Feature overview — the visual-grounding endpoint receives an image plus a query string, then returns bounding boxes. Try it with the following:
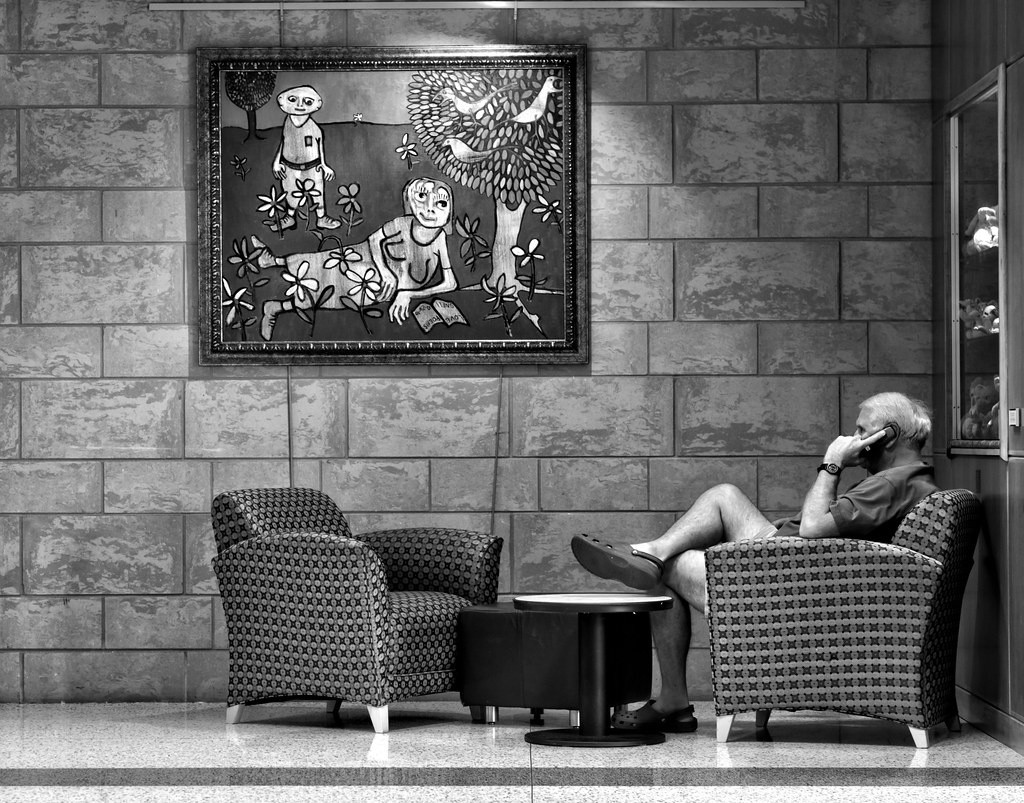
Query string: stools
[456,602,653,728]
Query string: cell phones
[858,427,895,459]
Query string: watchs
[816,462,841,475]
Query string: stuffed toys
[959,204,1012,441]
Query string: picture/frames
[195,43,591,367]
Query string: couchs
[211,487,504,735]
[705,489,982,749]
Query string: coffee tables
[512,593,674,748]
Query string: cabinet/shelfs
[936,62,1010,462]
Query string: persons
[572,392,937,733]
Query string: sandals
[571,532,664,590]
[611,699,698,732]
